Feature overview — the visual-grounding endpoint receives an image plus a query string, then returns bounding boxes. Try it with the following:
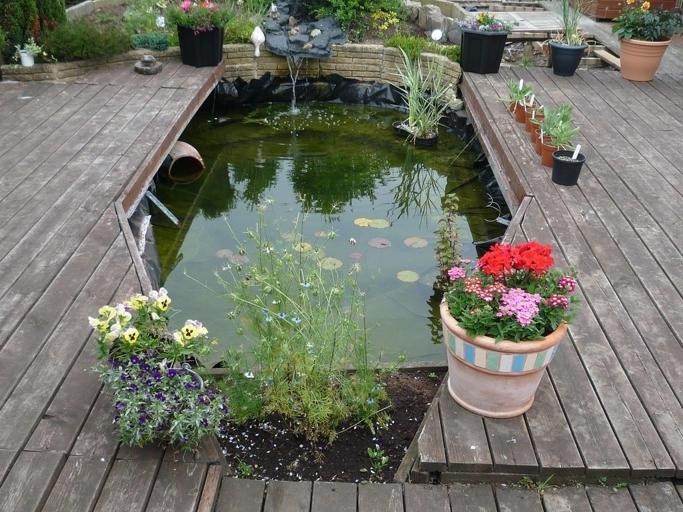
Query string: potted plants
[390,47,456,146]
[550,0,588,76]
[508,80,585,185]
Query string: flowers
[612,0,683,39]
[448,242,578,343]
[86,287,226,450]
[167,0,236,29]
[14,37,43,56]
[462,12,513,32]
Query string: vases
[439,296,566,419]
[177,24,224,67]
[461,30,508,74]
[620,37,672,81]
[19,50,34,67]
